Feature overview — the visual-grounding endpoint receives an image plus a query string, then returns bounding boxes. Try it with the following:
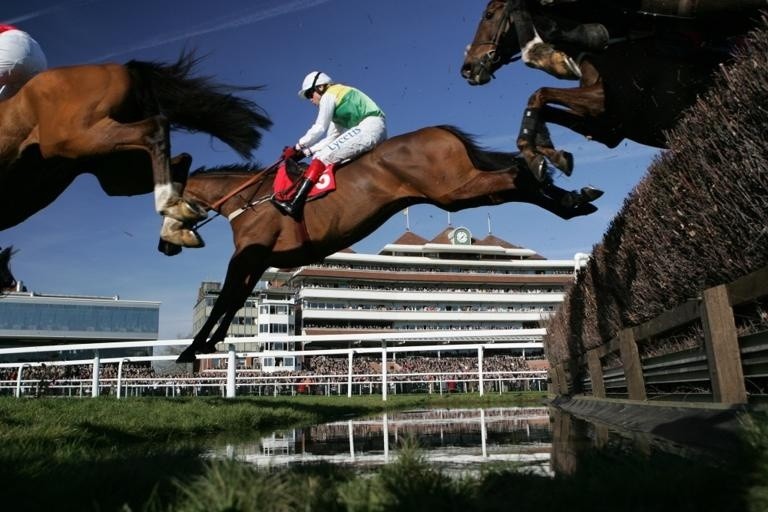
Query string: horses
[0,40,273,301]
[459,0,768,184]
[157,123,606,364]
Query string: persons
[0,20,49,97]
[269,70,387,224]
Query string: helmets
[297,71,333,98]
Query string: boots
[271,178,314,222]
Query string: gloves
[281,145,306,162]
